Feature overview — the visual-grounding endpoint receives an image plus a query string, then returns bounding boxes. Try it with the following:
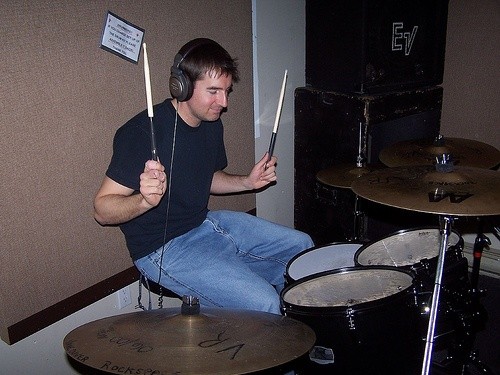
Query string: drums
[355,228,474,340]
[280,266,423,375]
[283,241,366,288]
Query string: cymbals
[318,163,386,189]
[64,296,317,375]
[352,167,500,216]
[378,135,500,168]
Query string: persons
[94,38,314,314]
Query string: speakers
[293,83,444,248]
[304,0,446,96]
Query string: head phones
[168,40,209,102]
